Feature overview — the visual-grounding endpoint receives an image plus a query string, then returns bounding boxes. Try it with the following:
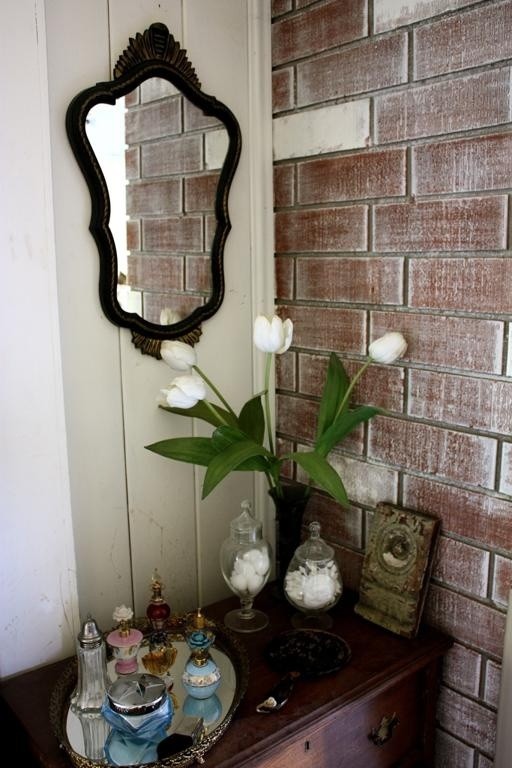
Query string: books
[354,500,441,640]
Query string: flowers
[149,318,422,498]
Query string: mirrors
[64,24,242,361]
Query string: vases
[265,484,310,594]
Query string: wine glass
[217,500,273,633]
[284,520,344,631]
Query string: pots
[255,629,351,716]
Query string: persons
[382,536,407,568]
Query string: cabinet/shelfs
[0,575,452,768]
